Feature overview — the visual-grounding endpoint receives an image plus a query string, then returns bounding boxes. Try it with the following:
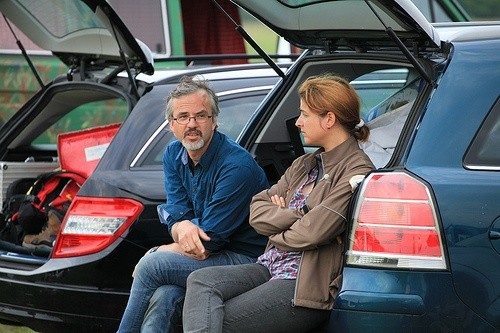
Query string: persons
[114,76,273,333]
[182,72,379,333]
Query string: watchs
[149,245,160,253]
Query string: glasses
[173,113,213,125]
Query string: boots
[22,209,63,249]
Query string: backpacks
[17,168,89,233]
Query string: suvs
[1,0,291,332]
[211,0,500,332]
